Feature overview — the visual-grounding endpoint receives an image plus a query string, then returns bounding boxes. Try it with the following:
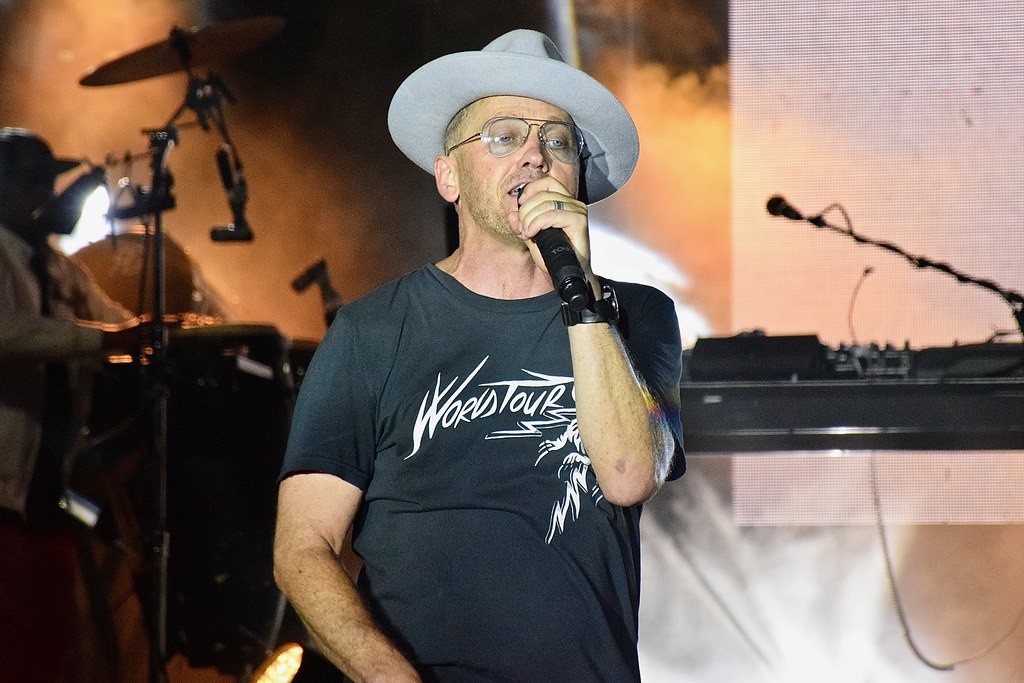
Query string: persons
[273,29,686,683]
[0,127,265,669]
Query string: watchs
[560,285,620,327]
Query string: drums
[65,230,227,328]
[159,322,295,454]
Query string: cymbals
[79,14,291,89]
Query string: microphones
[518,182,589,311]
[291,257,327,293]
[767,196,803,220]
[210,224,254,244]
[108,192,177,219]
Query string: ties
[30,256,51,318]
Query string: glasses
[447,115,585,164]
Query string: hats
[0,127,80,177]
[387,29,640,205]
